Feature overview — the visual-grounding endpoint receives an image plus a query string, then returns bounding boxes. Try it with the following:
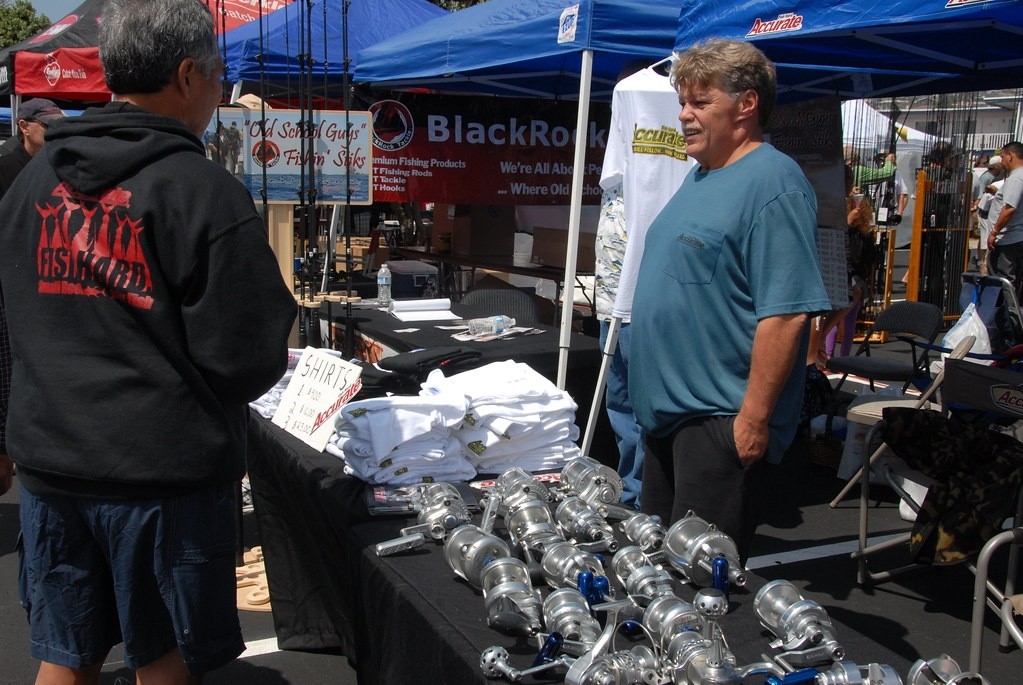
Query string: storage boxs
[531,227,597,272]
[337,242,389,272]
[452,203,516,256]
[384,260,438,298]
[837,399,953,489]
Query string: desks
[318,299,620,471]
[398,245,597,333]
[246,366,918,685]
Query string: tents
[579,0,1023,458]
[318,0,677,388]
[0,0,291,137]
[841,98,952,250]
[215,0,446,111]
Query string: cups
[854,194,864,208]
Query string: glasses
[25,119,49,129]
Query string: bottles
[377,264,391,305]
[470,314,516,335]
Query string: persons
[844,149,908,296]
[596,181,647,508]
[902,142,1023,305]
[0,0,297,685]
[0,99,66,494]
[628,42,833,569]
[203,122,244,177]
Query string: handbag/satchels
[929,284,993,374]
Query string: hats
[989,156,1002,165]
[15,99,65,126]
[843,146,858,165]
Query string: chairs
[463,288,536,321]
[824,273,1023,671]
